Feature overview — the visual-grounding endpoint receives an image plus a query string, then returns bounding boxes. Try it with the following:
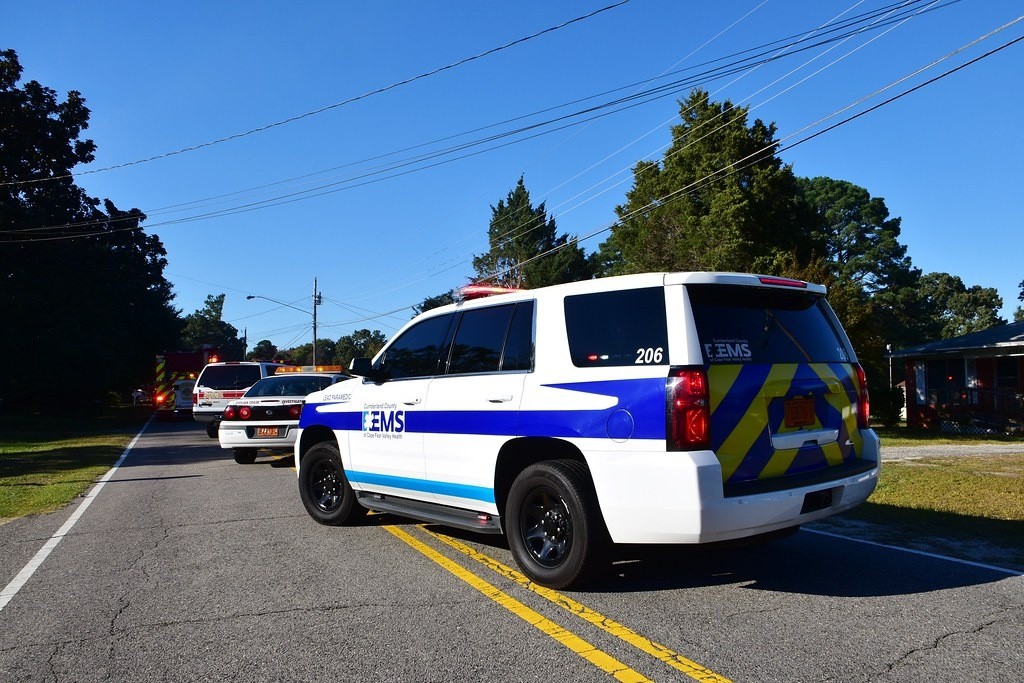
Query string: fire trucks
[146,343,224,423]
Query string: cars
[218,365,352,464]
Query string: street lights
[247,295,317,367]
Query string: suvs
[191,359,288,439]
[293,273,882,593]
